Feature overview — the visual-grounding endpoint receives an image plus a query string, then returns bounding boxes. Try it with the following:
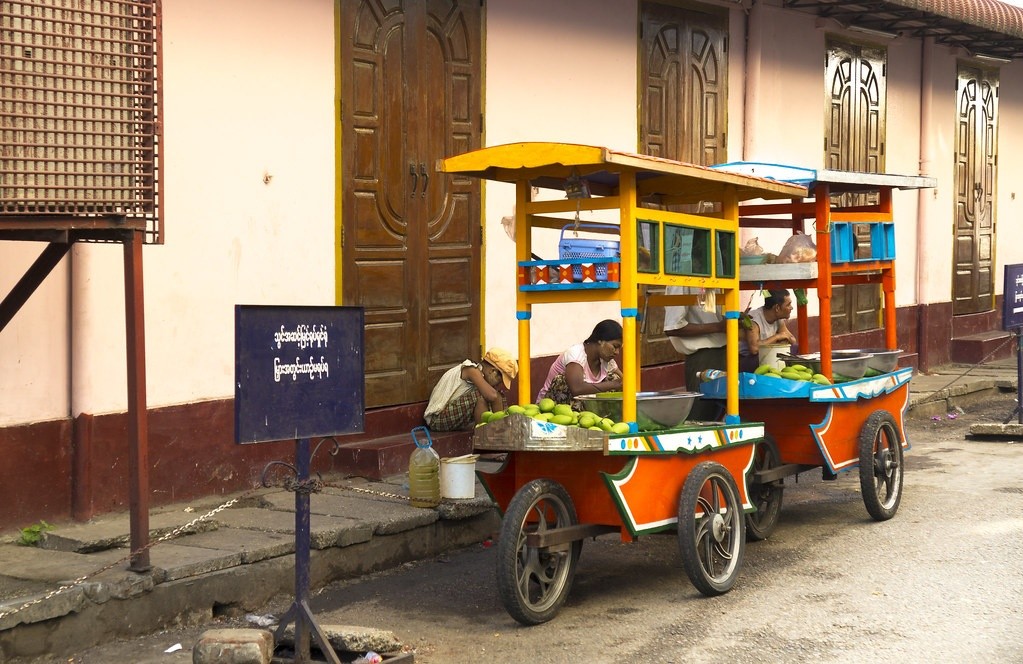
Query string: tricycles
[691,148,938,522]
[435,141,768,628]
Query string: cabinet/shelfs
[493,166,742,428]
[735,183,897,377]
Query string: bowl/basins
[829,348,905,377]
[738,254,768,266]
[573,391,704,432]
[784,351,874,384]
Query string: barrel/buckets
[440,457,476,499]
[757,344,791,373]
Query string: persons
[535,318,622,413]
[745,289,798,372]
[424,346,518,461]
[665,286,748,423]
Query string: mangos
[475,398,630,434]
[753,364,831,384]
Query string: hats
[483,346,518,390]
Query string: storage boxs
[558,224,622,281]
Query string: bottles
[409,426,441,508]
[696,369,727,382]
